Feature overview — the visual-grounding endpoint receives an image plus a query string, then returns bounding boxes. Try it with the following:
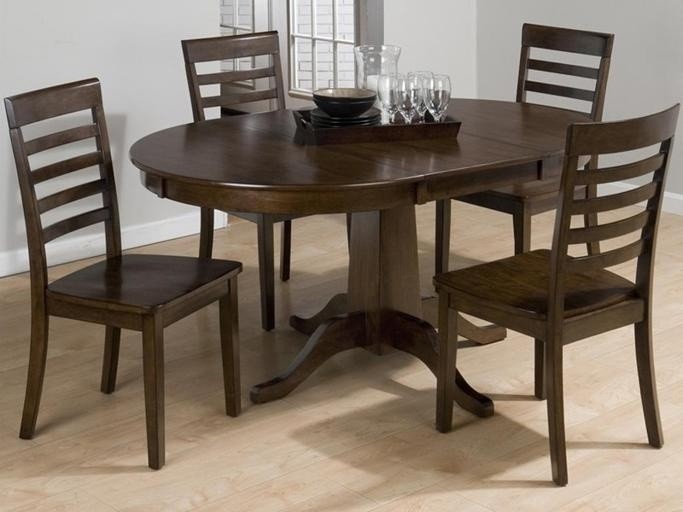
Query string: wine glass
[376,70,453,124]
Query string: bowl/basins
[311,87,378,118]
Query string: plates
[309,106,383,130]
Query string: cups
[353,45,402,127]
